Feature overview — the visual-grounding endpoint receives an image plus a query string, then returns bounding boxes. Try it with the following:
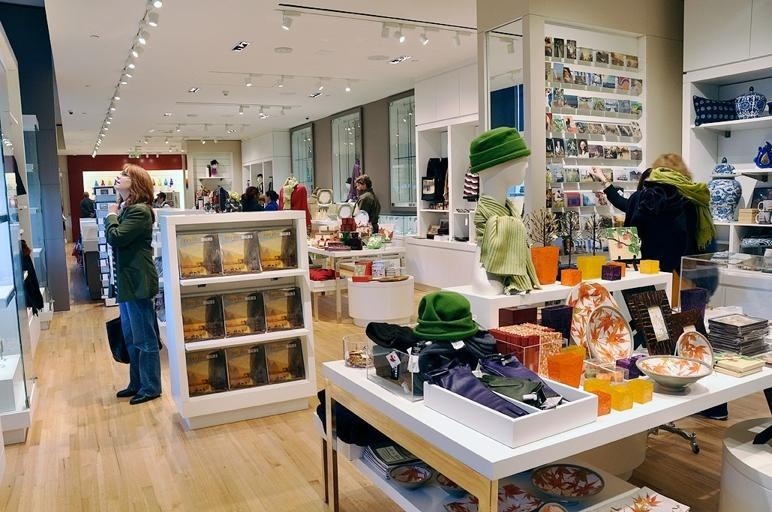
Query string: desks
[308,246,407,323]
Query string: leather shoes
[130,392,160,405]
[117,388,140,397]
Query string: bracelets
[601,178,609,185]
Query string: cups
[755,199,772,223]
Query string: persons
[263,190,281,211]
[467,125,541,298]
[554,139,565,158]
[80,191,97,218]
[622,152,728,421]
[587,165,652,227]
[276,176,312,227]
[257,173,263,193]
[561,68,572,83]
[353,172,380,237]
[240,186,264,212]
[345,178,354,204]
[576,139,588,159]
[102,161,163,406]
[154,190,170,210]
[553,189,563,204]
[210,159,220,178]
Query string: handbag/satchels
[106,312,162,364]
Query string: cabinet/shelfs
[682,0,772,322]
[402,63,479,293]
[0,23,54,512]
[159,209,318,430]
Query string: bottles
[95,179,117,186]
[206,165,211,178]
[152,178,174,188]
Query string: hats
[413,291,478,340]
[470,127,531,174]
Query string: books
[702,313,771,379]
[360,441,429,480]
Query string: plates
[566,279,620,348]
[637,354,714,393]
[531,461,607,502]
[586,305,634,366]
[435,473,467,500]
[588,485,692,512]
[438,482,539,512]
[388,464,432,491]
[539,501,569,512]
[676,331,715,371]
